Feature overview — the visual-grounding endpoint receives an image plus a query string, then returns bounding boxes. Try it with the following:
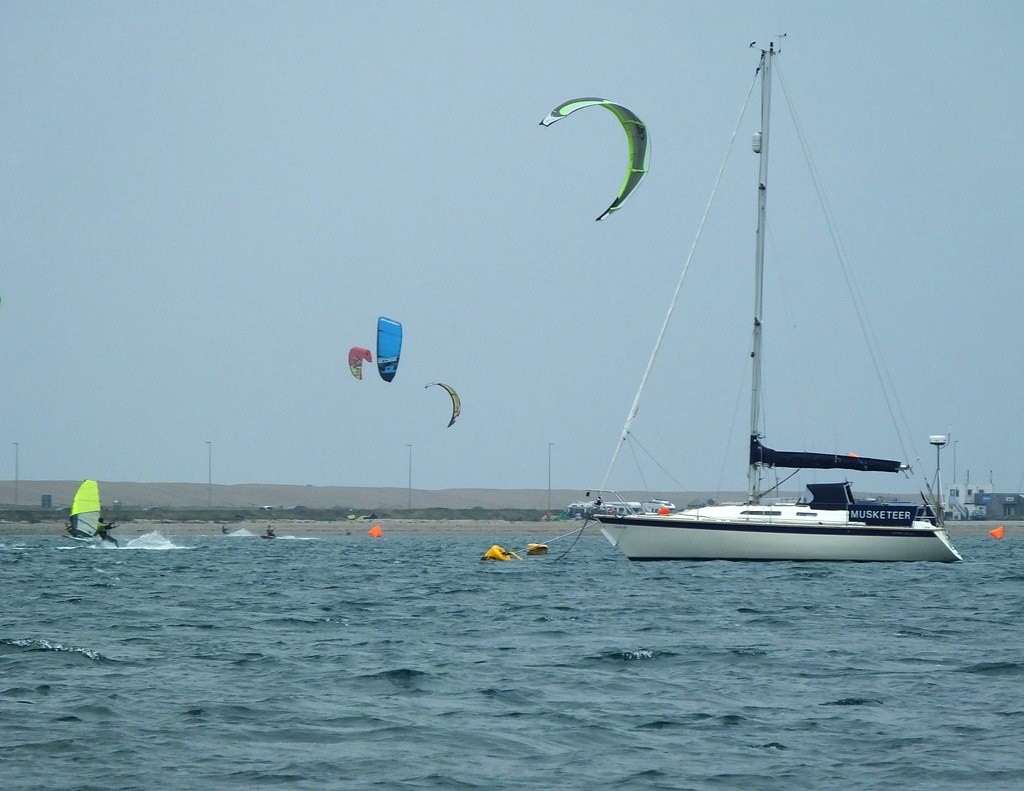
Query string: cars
[970,509,987,521]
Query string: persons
[97,517,120,548]
[267,525,276,536]
[222,525,229,534]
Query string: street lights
[407,444,411,516]
[11,442,18,505]
[547,442,555,518]
[205,441,211,510]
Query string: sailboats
[60,479,122,548]
[589,28,965,564]
